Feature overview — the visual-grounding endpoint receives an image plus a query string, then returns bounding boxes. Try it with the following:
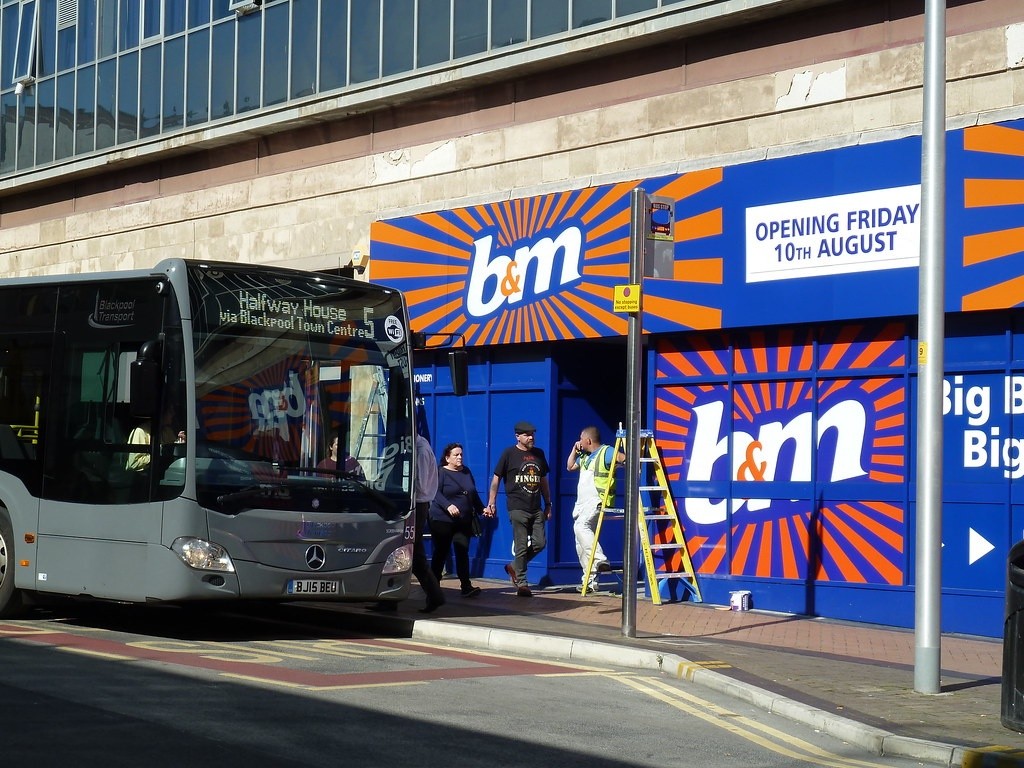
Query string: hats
[514,422,536,432]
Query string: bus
[0,256,469,620]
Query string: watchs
[546,502,552,507]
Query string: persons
[365,432,447,613]
[426,442,496,597]
[125,393,186,500]
[567,427,626,592]
[483,422,552,598]
[316,433,367,480]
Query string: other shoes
[365,600,397,612]
[461,586,481,597]
[518,586,532,597]
[418,601,444,613]
[577,585,593,593]
[504,564,519,587]
[599,564,613,575]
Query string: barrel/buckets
[729,590,751,611]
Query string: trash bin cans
[1001,539,1024,733]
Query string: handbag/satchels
[471,512,482,537]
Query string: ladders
[581,428,703,606]
